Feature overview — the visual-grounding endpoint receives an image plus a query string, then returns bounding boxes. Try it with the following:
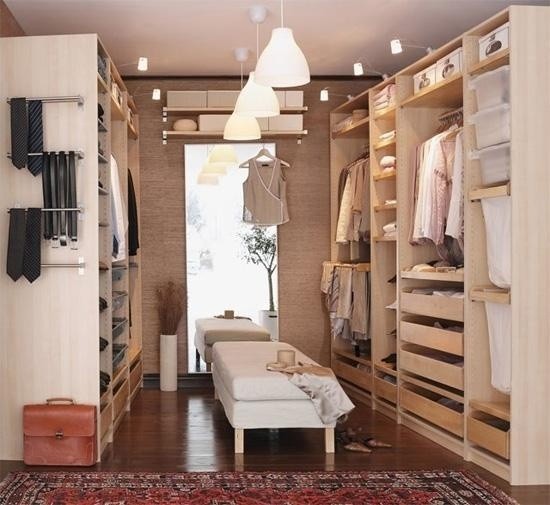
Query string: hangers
[238,144,292,171]
[346,145,368,166]
[413,107,463,148]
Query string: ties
[27,100,43,177]
[7,208,26,281]
[22,207,41,283]
[11,97,27,169]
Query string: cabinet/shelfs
[0,34,145,463]
[162,104,307,138]
[328,5,549,485]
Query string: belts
[42,151,51,246]
[50,151,59,248]
[59,151,67,246]
[68,151,78,250]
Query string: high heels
[345,426,392,447]
[335,426,372,452]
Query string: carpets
[0,471,523,504]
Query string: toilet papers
[278,350,295,366]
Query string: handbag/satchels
[23,397,98,467]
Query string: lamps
[116,57,148,74]
[233,6,281,118]
[353,63,386,79]
[132,88,161,101]
[320,88,352,101]
[254,0,311,88]
[390,39,434,54]
[223,48,262,139]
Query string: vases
[158,333,178,393]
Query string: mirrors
[183,144,279,374]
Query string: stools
[213,342,355,455]
[194,315,272,371]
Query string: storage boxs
[478,21,509,61]
[436,47,463,81]
[413,62,436,93]
[467,66,510,184]
